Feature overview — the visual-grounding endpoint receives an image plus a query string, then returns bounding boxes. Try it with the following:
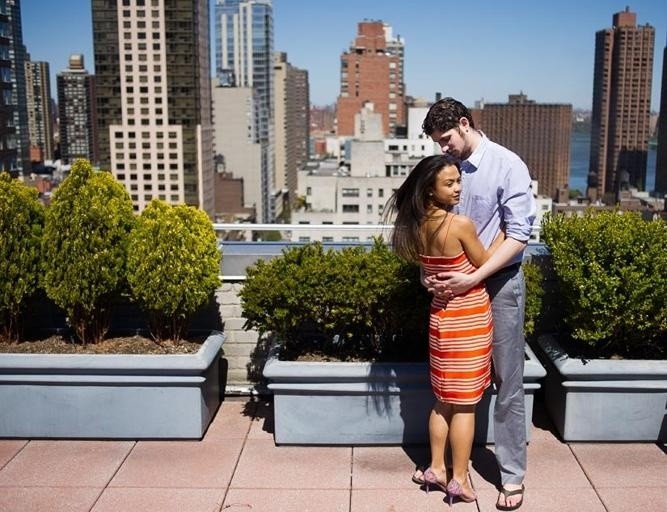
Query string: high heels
[446,478,477,507]
[423,467,452,494]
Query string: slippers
[412,464,430,484]
[496,484,525,511]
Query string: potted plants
[0,159,227,442]
[237,235,548,448]
[536,188,666,445]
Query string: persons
[377,153,504,505]
[421,95,537,511]
